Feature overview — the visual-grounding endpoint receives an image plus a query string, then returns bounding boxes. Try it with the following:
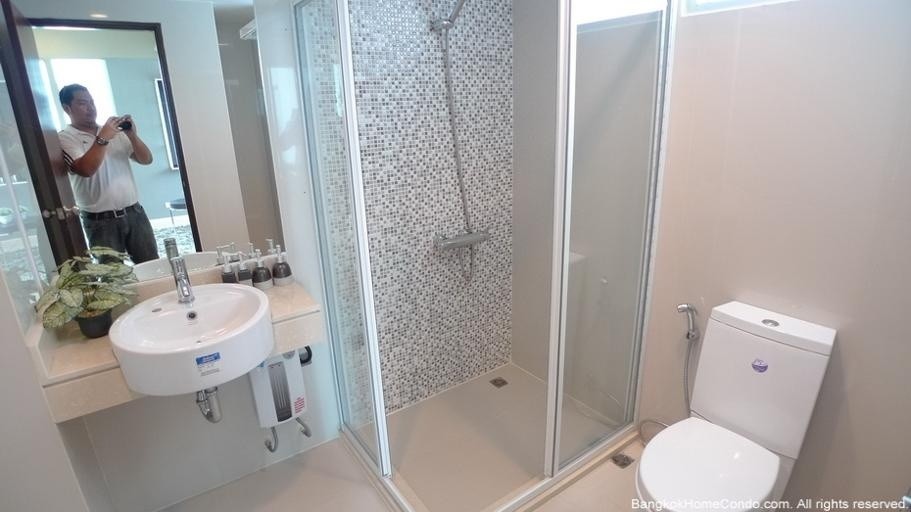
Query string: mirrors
[0,1,288,290]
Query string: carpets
[149,225,197,258]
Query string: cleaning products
[222,245,293,289]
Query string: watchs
[96,135,110,146]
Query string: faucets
[170,257,193,304]
[164,238,178,263]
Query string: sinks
[123,251,234,281]
[108,284,273,398]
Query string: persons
[56,84,161,266]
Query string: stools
[166,196,188,225]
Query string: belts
[80,204,142,221]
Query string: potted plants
[34,245,139,340]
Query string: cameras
[116,120,134,132]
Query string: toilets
[635,302,839,512]
